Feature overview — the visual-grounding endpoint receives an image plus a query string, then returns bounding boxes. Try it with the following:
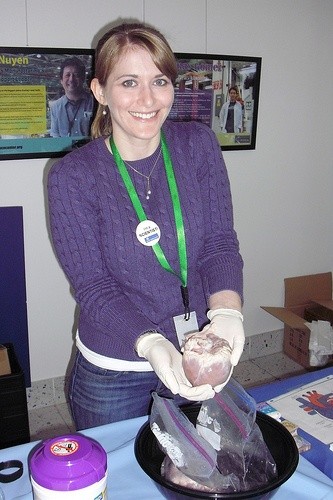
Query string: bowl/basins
[133,404,300,500]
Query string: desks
[0,368,332,500]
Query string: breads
[183,334,232,385]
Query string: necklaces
[123,149,162,200]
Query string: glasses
[63,72,82,78]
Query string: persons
[48,55,95,136]
[46,22,246,431]
[220,86,242,133]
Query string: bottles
[26,434,109,500]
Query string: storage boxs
[257,273,333,372]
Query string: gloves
[181,307,244,393]
[135,333,217,400]
